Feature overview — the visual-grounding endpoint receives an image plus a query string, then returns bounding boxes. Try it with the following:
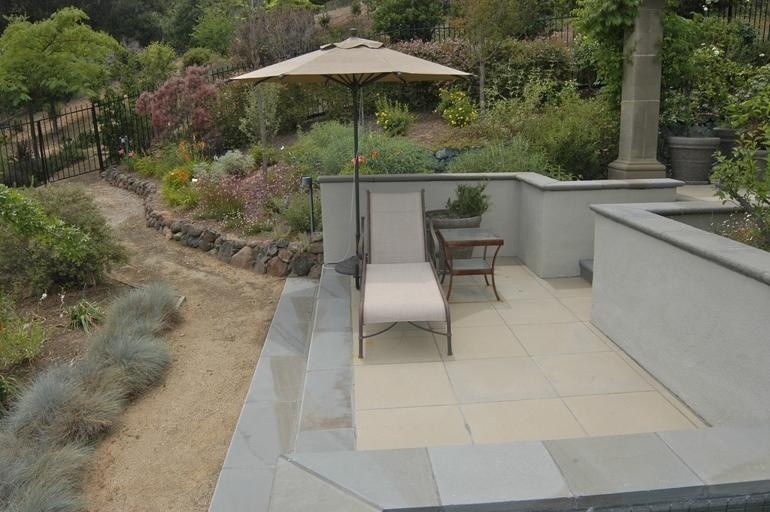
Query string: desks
[437,227,503,301]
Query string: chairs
[358,188,452,358]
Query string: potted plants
[426,180,493,274]
[666,92,770,184]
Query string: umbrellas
[223,27,479,289]
